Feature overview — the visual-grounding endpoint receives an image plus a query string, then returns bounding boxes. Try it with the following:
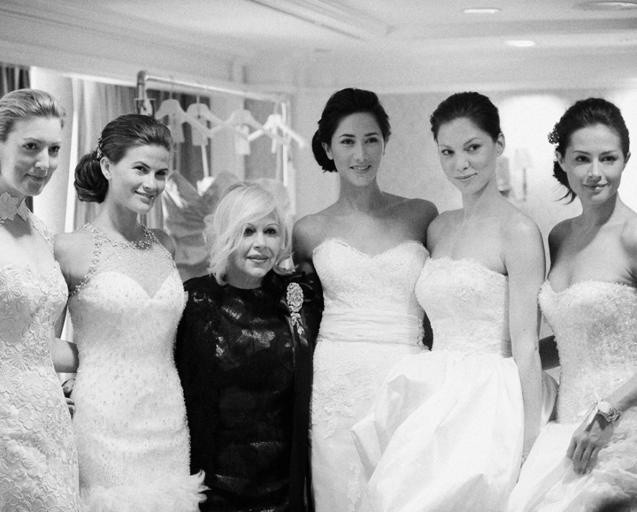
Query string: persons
[172,180,323,511]
[50,112,211,512]
[292,86,440,511]
[499,96,636,512]
[0,86,81,512]
[355,90,561,512]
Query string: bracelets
[592,396,622,424]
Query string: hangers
[152,102,307,160]
